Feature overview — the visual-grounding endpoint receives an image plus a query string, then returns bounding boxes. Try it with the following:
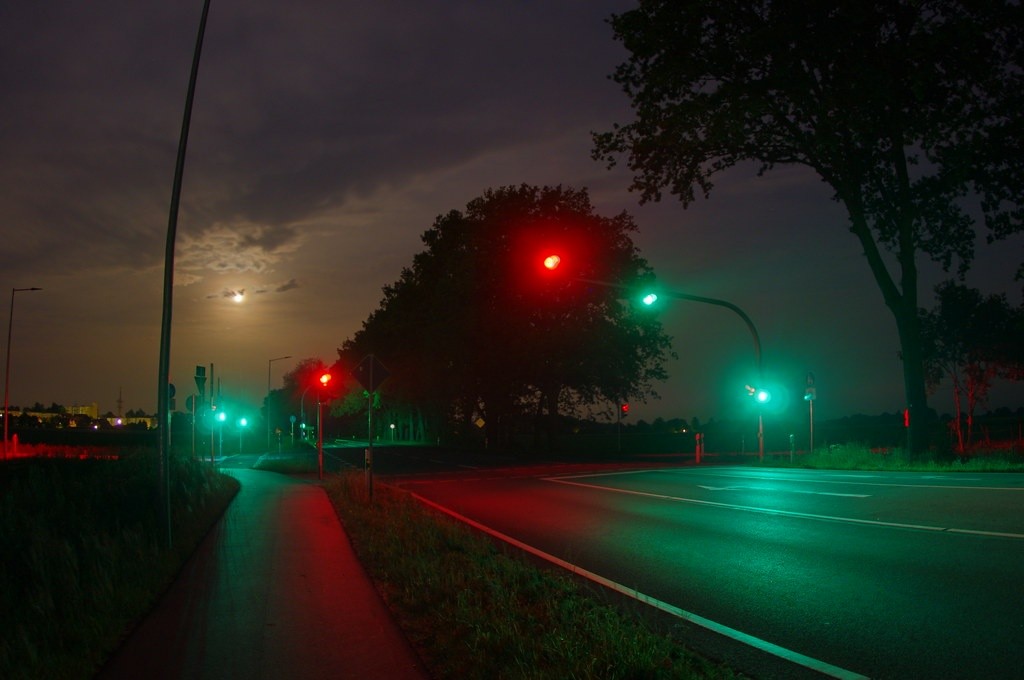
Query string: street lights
[3,286,45,461]
[267,355,293,453]
